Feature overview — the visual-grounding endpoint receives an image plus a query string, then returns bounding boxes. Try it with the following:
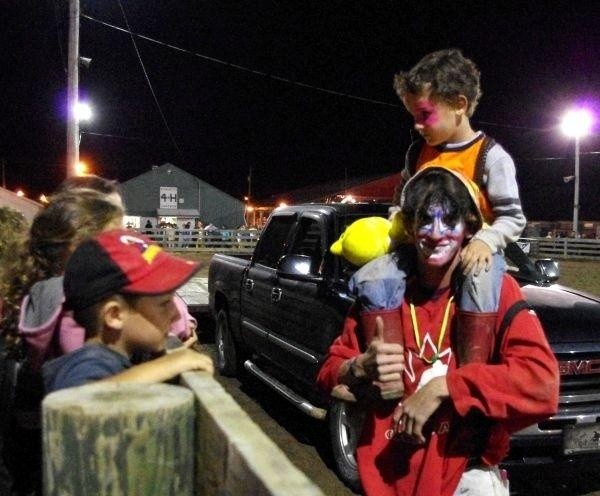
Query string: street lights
[561,105,592,238]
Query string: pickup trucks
[207,200,599,494]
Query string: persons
[6,231,207,493]
[62,175,123,208]
[18,193,198,356]
[348,50,527,401]
[317,166,559,496]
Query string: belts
[463,456,490,472]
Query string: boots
[356,308,406,402]
[453,308,500,370]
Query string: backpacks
[0,302,70,493]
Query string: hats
[60,227,202,312]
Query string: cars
[182,228,260,252]
